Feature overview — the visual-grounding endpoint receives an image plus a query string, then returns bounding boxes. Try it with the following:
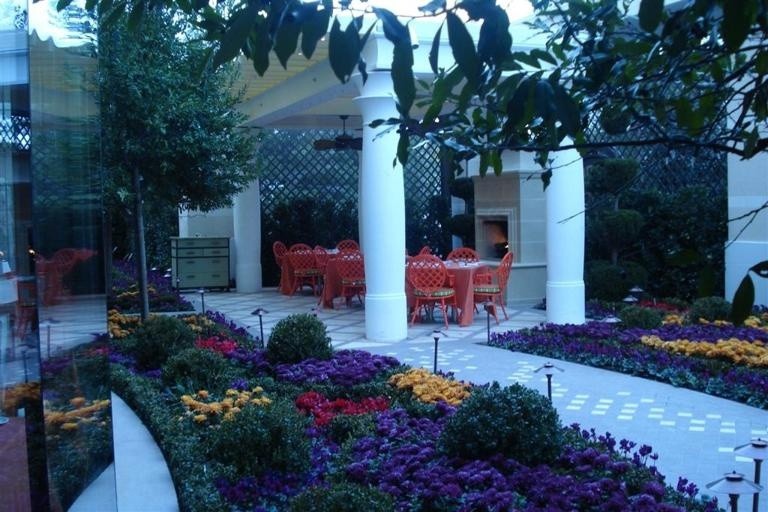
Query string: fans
[314,115,362,150]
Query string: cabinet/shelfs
[169,237,230,294]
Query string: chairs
[273,239,514,330]
[6,250,95,342]
